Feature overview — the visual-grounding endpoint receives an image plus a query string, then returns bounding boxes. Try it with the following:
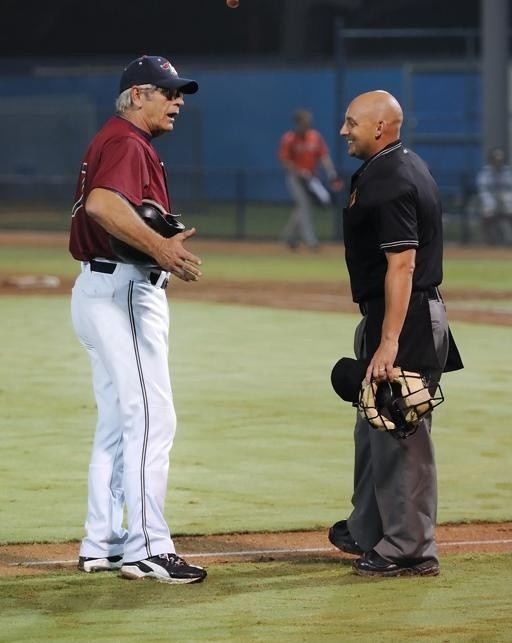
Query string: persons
[326,87,453,579]
[275,107,345,254]
[463,147,512,247]
[66,54,208,588]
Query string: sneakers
[328,519,369,555]
[78,550,125,573]
[122,555,209,586]
[353,542,440,580]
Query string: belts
[90,258,169,290]
[358,285,442,316]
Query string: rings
[379,368,386,372]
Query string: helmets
[358,365,445,440]
[108,199,185,266]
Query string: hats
[119,55,198,95]
